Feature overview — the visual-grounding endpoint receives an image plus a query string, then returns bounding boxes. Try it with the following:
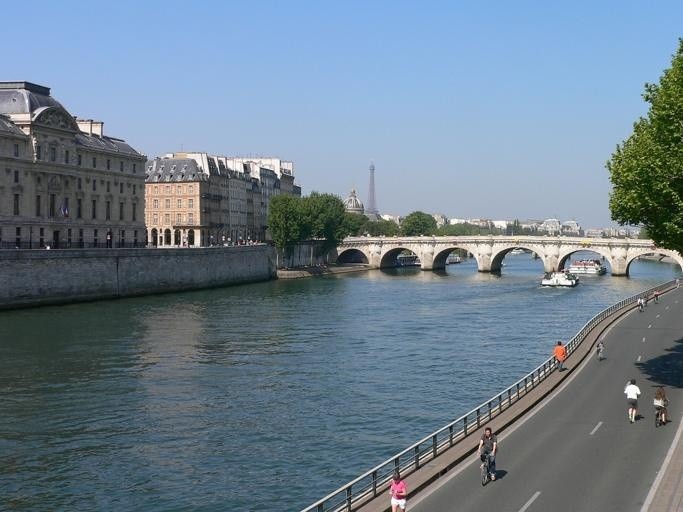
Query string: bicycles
[478,453,499,485]
[638,296,659,312]
[654,396,672,427]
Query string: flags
[60,205,71,218]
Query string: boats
[532,252,539,259]
[397,254,463,267]
[539,268,579,287]
[567,260,607,275]
[510,249,525,254]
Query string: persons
[389,471,407,512]
[597,340,605,362]
[637,288,662,312]
[208,233,260,247]
[676,278,680,289]
[553,341,568,372]
[653,386,669,425]
[624,379,642,424]
[476,427,497,481]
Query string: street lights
[105,232,112,249]
[209,239,262,247]
